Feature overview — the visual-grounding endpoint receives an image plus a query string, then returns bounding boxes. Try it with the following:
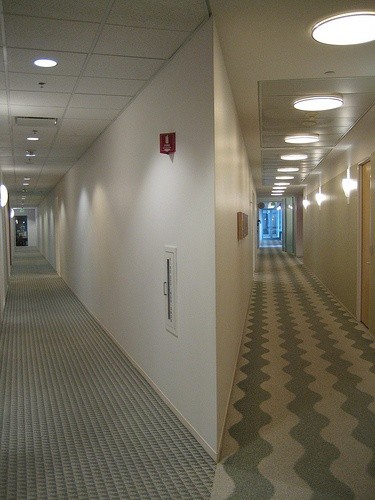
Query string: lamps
[10,208,14,218]
[285,133,319,144]
[303,196,310,208]
[271,168,299,195]
[342,169,358,204]
[280,154,308,160]
[315,187,326,206]
[312,10,375,45]
[0,185,8,207]
[293,95,343,111]
